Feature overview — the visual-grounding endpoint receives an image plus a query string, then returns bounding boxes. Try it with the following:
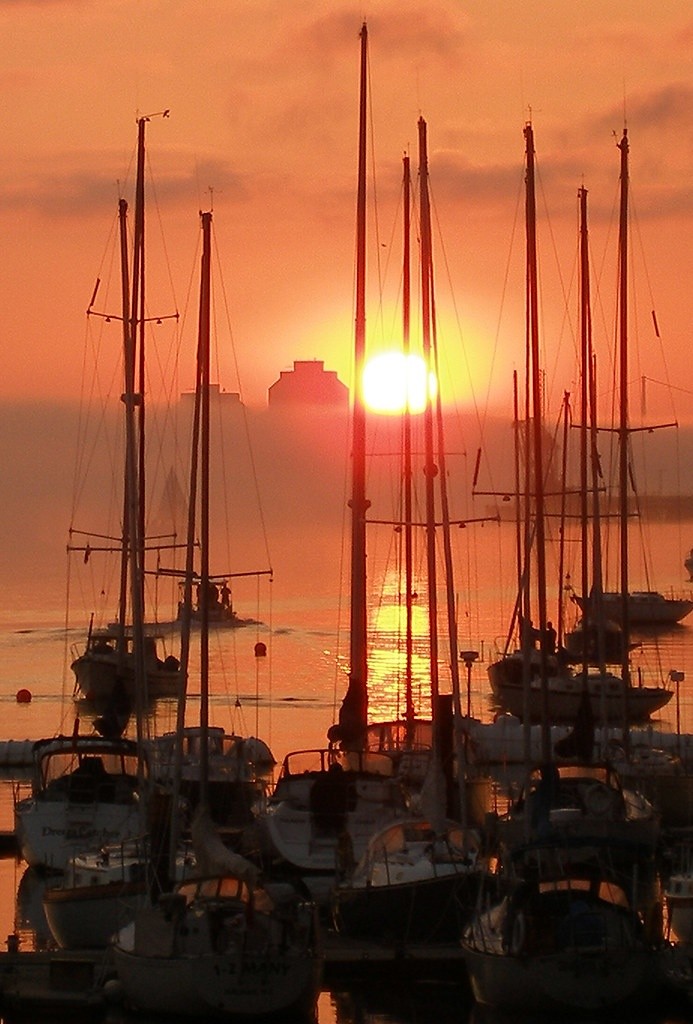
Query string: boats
[0,714,693,1024]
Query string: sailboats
[327,19,693,798]
[155,186,272,625]
[60,108,186,705]
